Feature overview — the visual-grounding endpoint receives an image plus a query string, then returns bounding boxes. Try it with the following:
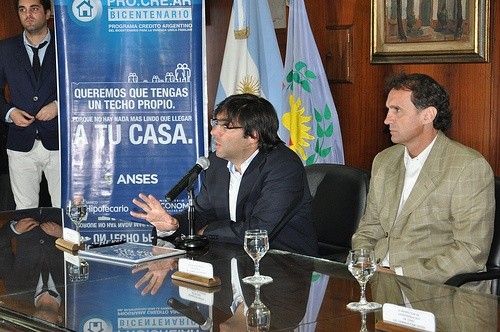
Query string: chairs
[304,163,371,261]
[445,180,500,299]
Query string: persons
[132,246,314,332]
[345,74,495,295]
[0,207,64,325]
[366,271,498,332]
[0,0,61,209]
[131,93,319,257]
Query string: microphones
[165,156,210,203]
[167,297,213,330]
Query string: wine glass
[68,259,89,283]
[350,309,376,332]
[241,230,273,282]
[245,282,270,332]
[346,249,383,309]
[66,198,91,241]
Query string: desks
[0,207,500,332]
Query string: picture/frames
[369,0,489,63]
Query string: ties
[28,41,48,82]
[34,265,58,298]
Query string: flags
[210,0,345,166]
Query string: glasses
[210,118,244,130]
